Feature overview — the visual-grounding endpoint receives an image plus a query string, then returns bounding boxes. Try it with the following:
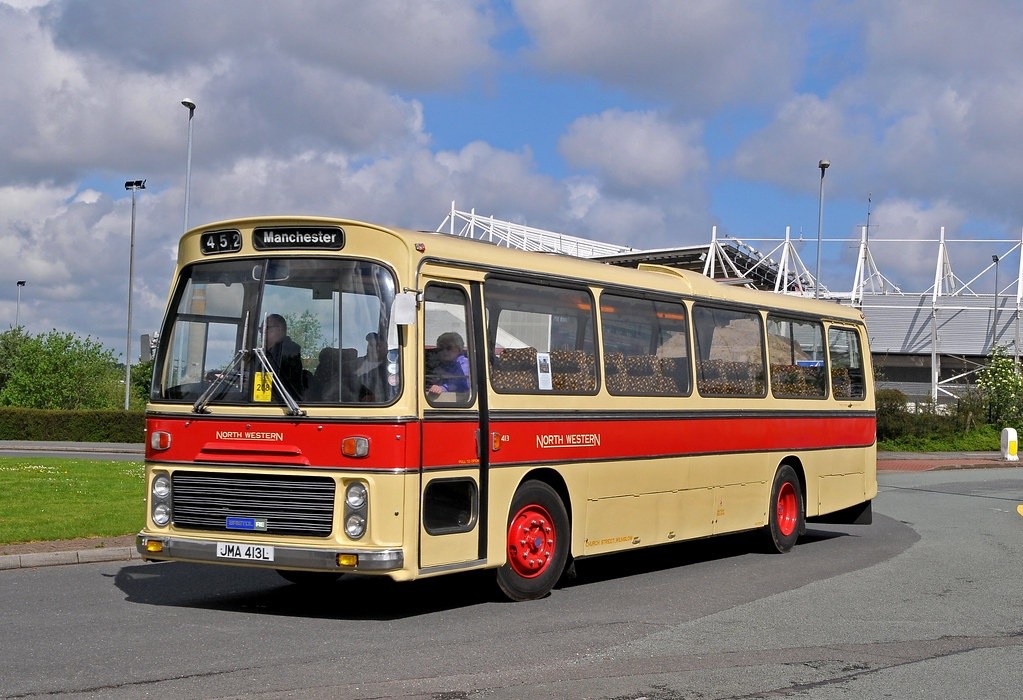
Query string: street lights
[177,98,196,384]
[992,254,999,348]
[123,179,148,410]
[812,158,830,362]
[16,279,25,329]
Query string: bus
[134,216,879,602]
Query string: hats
[366,332,387,345]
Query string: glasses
[437,344,457,352]
[368,341,385,348]
[256,324,280,333]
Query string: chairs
[308,346,851,404]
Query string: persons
[228,314,304,403]
[429,332,471,394]
[333,332,396,402]
